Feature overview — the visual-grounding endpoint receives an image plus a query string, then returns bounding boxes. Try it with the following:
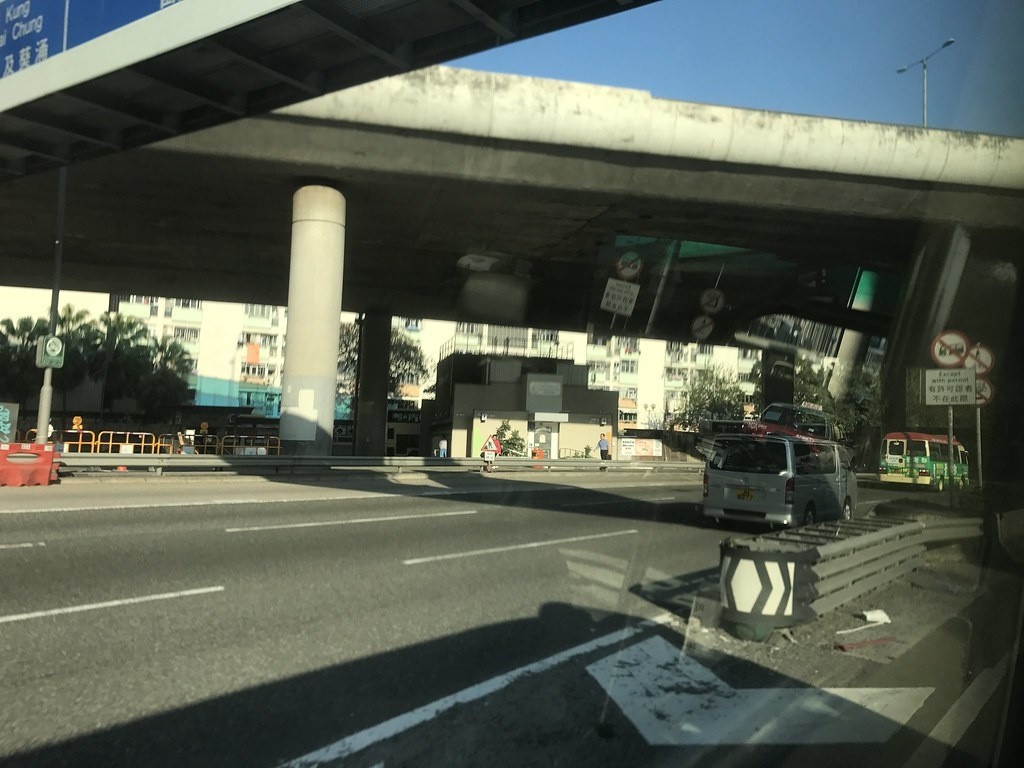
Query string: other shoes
[492,466,498,469]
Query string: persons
[493,435,501,456]
[48,419,54,441]
[594,433,609,471]
[439,437,447,457]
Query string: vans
[882,431,971,490]
[702,418,749,433]
[702,432,859,532]
[759,402,848,448]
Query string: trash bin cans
[532,448,543,468]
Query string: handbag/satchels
[606,455,611,459]
